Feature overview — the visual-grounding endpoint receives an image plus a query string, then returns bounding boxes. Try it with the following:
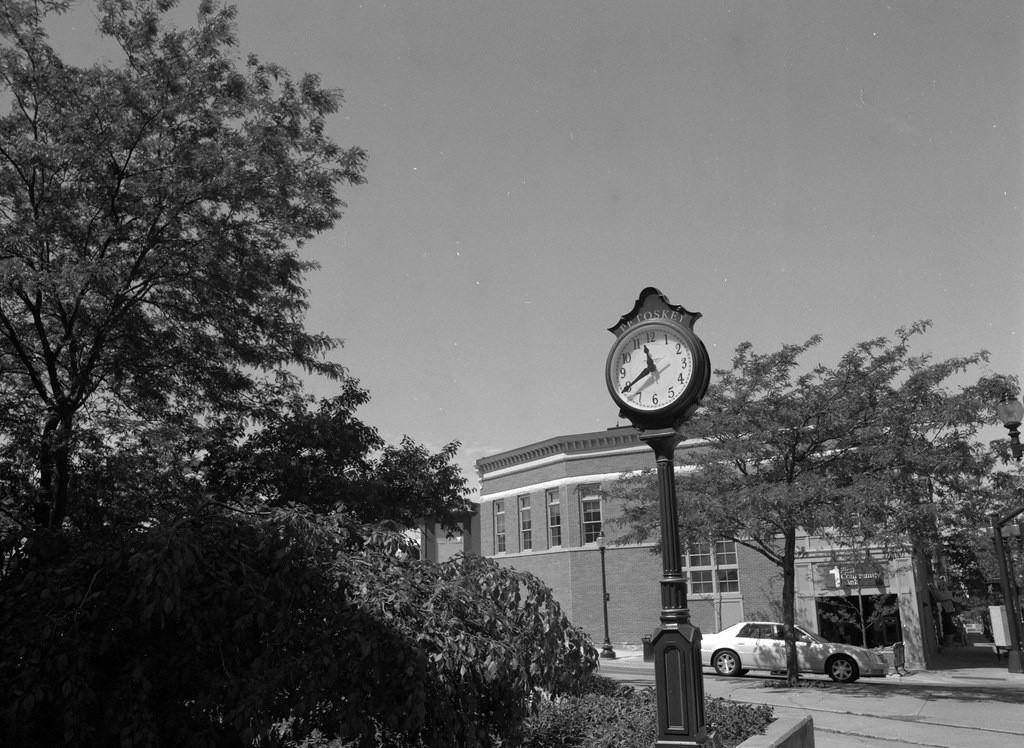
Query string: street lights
[987,509,1024,648]
[597,530,616,658]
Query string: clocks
[603,318,702,419]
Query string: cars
[701,621,890,682]
